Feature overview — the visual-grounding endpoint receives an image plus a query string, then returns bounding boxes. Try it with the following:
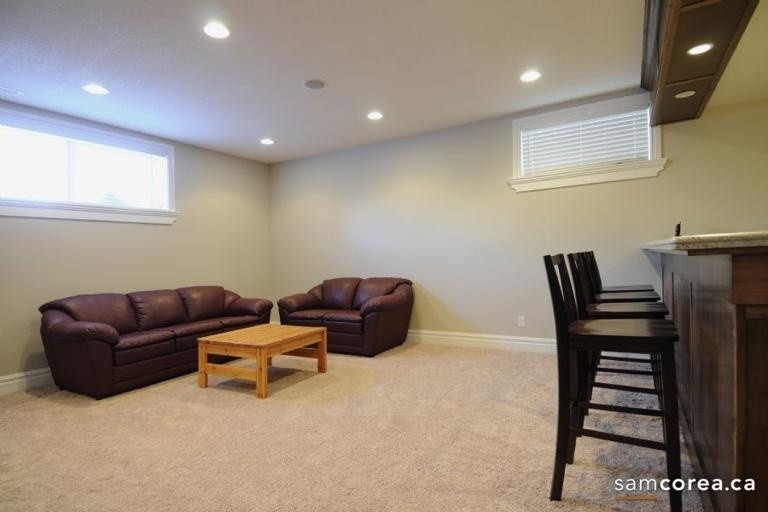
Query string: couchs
[38,290,273,399]
[277,283,414,357]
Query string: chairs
[543,252,682,512]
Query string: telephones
[674,221,682,237]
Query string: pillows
[127,289,186,331]
[176,286,225,321]
[354,278,412,309]
[323,277,360,310]
[39,294,138,334]
